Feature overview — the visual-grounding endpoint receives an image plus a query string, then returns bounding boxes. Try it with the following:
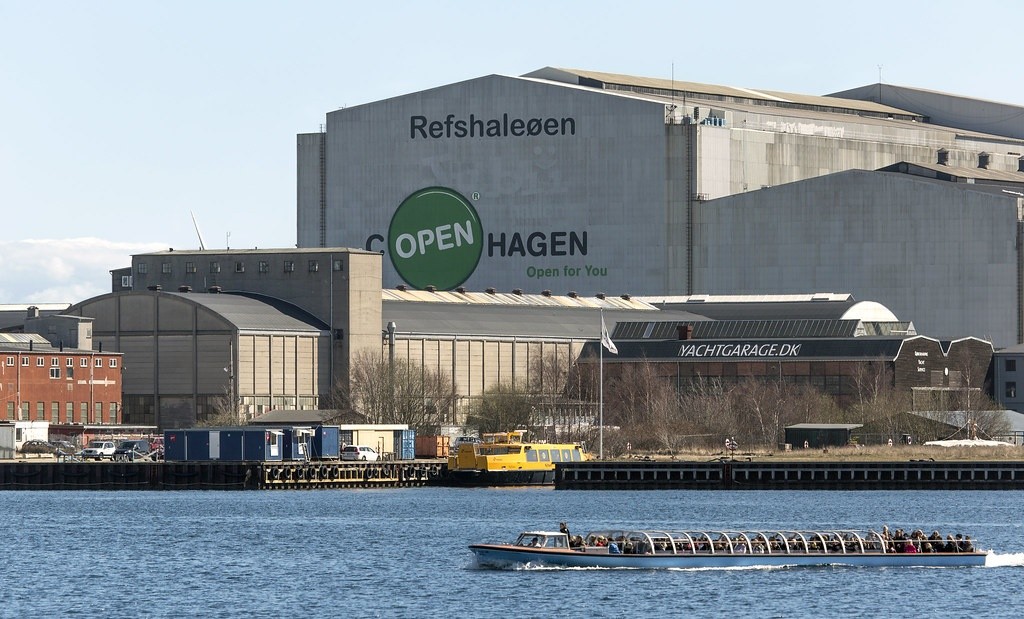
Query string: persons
[560,522,973,554]
[529,538,541,547]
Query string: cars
[51,441,75,453]
[22,439,57,453]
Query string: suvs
[81,441,115,461]
[144,437,165,451]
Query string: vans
[113,441,150,459]
[341,445,378,461]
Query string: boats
[430,429,591,486]
[469,528,989,570]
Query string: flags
[600,314,619,354]
